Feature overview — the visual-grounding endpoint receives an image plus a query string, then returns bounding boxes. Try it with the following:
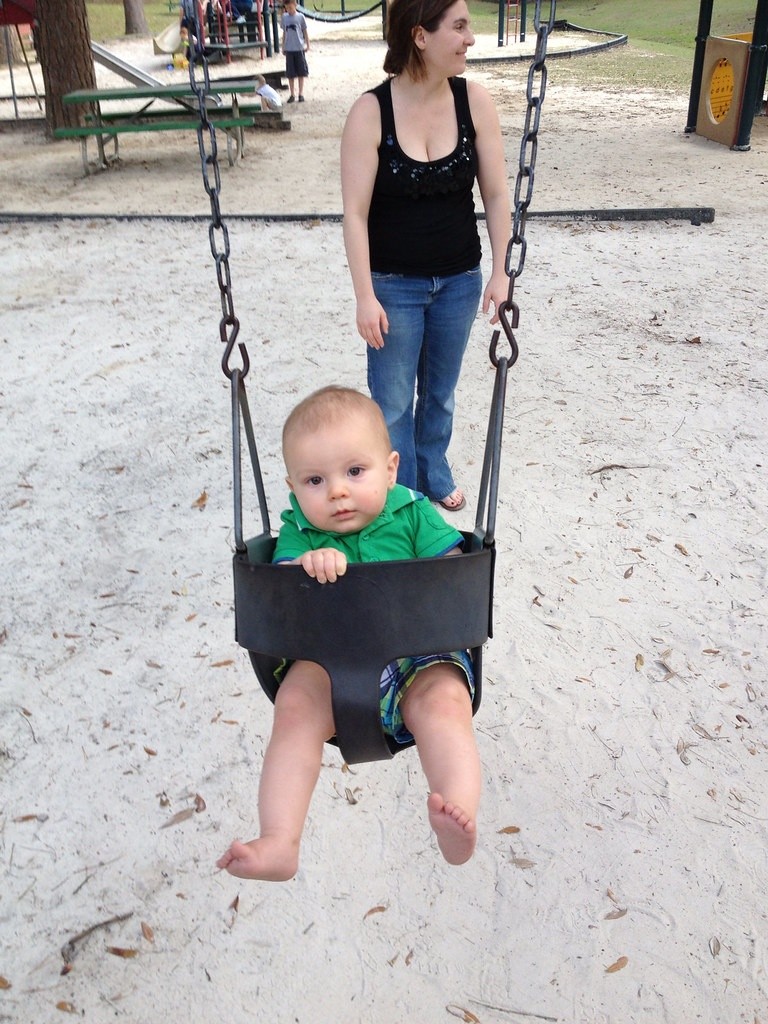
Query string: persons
[172,0,256,68]
[339,1,512,511]
[217,386,480,880]
[253,75,282,112]
[280,0,309,102]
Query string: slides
[153,19,183,56]
[89,40,224,110]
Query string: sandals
[437,486,466,510]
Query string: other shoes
[299,96,304,101]
[287,96,295,102]
[226,11,233,17]
[236,16,246,24]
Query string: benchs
[49,117,261,175]
[83,102,260,162]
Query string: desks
[62,80,259,171]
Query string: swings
[179,0,560,761]
